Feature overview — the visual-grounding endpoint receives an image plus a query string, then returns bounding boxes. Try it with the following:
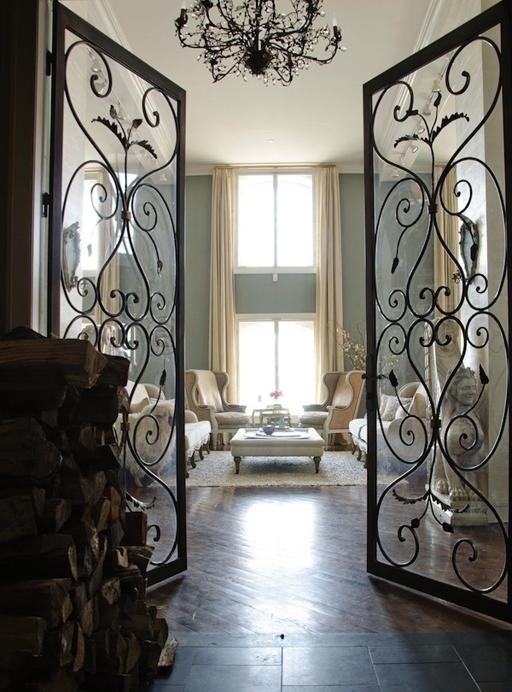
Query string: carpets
[145,450,410,488]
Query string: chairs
[185,368,368,450]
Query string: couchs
[349,381,439,476]
[111,379,213,488]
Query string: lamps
[174,0,346,86]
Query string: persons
[445,367,480,415]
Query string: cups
[263,425,274,436]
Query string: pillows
[378,392,412,421]
[130,395,176,417]
